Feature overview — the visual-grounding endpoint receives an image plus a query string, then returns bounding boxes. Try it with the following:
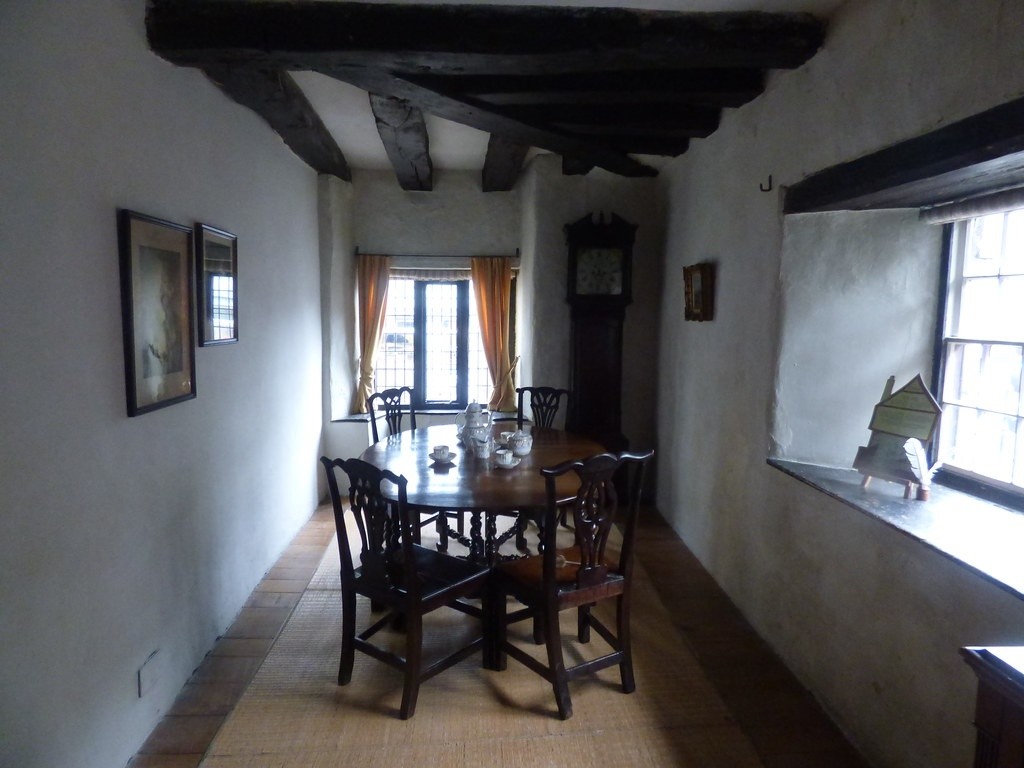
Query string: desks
[358,425,608,647]
[959,645,1024,768]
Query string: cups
[501,432,514,445]
[509,430,533,456]
[433,445,449,459]
[496,449,513,465]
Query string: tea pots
[454,400,492,453]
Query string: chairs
[366,387,465,554]
[491,449,655,720]
[320,456,491,719]
[498,386,574,552]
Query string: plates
[429,452,457,464]
[495,457,522,469]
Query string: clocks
[562,205,638,301]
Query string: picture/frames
[196,221,241,345]
[117,207,196,418]
[683,262,715,321]
